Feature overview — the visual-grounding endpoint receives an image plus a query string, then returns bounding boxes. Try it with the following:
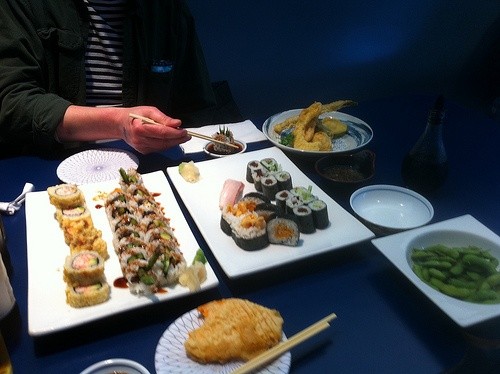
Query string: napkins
[179,119,267,154]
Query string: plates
[166,147,375,280]
[371,214,500,329]
[154,309,292,374]
[262,108,373,160]
[56,148,139,186]
[23,170,220,337]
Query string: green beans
[410,244,500,307]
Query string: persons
[0,0,214,154]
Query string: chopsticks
[129,112,239,149]
[230,312,337,374]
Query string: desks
[0,95,500,374]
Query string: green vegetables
[280,119,332,146]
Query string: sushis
[218,158,329,251]
[106,166,188,297]
[46,183,111,308]
[213,126,235,153]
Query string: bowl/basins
[79,358,150,374]
[315,150,376,196]
[349,184,434,237]
[203,139,247,159]
